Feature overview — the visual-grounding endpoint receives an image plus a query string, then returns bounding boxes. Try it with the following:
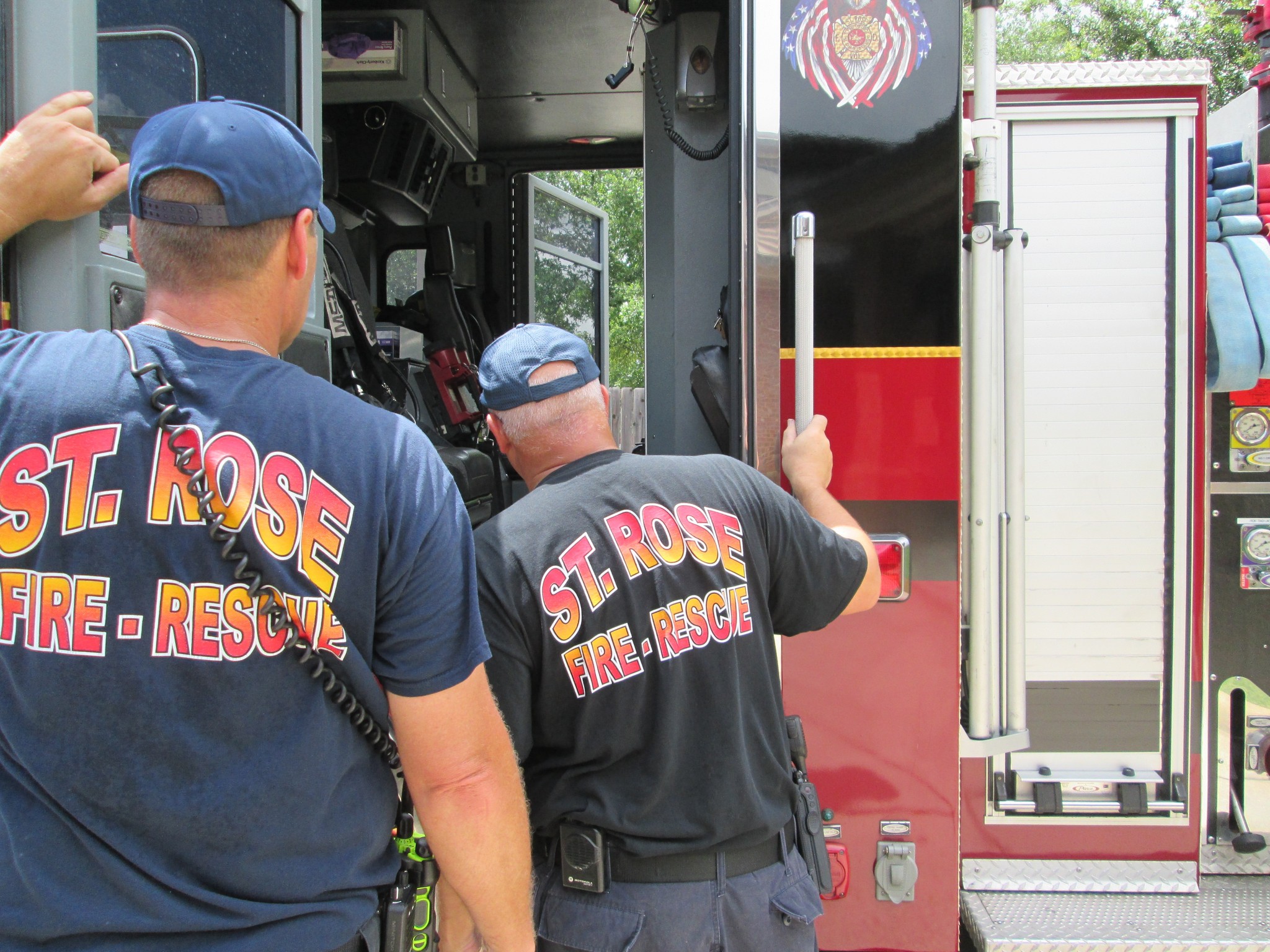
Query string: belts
[608,822,796,884]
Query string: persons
[0,88,535,952]
[437,324,880,952]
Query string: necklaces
[138,321,275,359]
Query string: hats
[130,96,336,235]
[477,321,600,410]
